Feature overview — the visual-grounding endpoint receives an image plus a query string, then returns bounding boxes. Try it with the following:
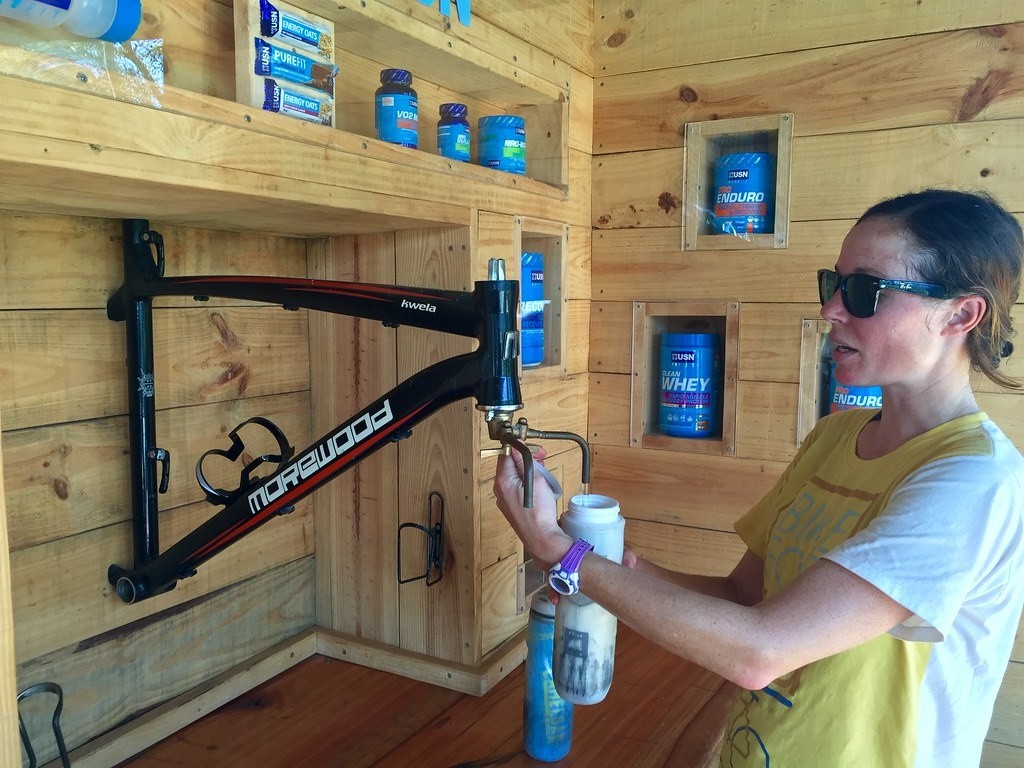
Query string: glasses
[816,266,950,316]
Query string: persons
[493,190,1024,767]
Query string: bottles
[0,0,143,44]
[437,103,471,162]
[524,592,574,763]
[552,492,628,705]
[375,68,419,148]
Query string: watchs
[547,538,595,596]
